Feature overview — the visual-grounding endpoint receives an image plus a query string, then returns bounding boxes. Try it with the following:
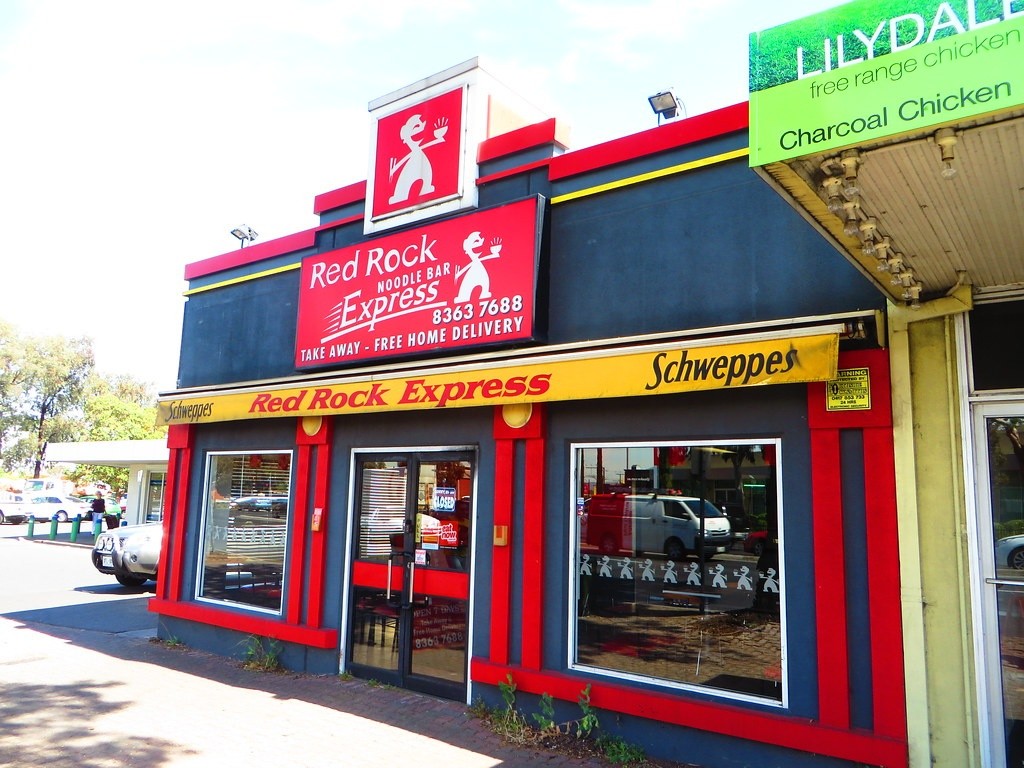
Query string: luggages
[104,508,120,529]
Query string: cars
[220,492,287,516]
[91,520,166,587]
[25,494,93,521]
[361,505,442,536]
[79,495,121,518]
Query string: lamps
[843,196,861,239]
[821,176,843,216]
[873,237,890,274]
[840,148,867,202]
[887,253,902,287]
[933,126,965,181]
[900,267,912,300]
[648,87,688,126]
[910,281,923,310]
[231,223,259,249]
[859,218,877,258]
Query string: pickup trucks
[0,493,34,526]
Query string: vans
[587,487,731,561]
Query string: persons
[89,491,106,535]
